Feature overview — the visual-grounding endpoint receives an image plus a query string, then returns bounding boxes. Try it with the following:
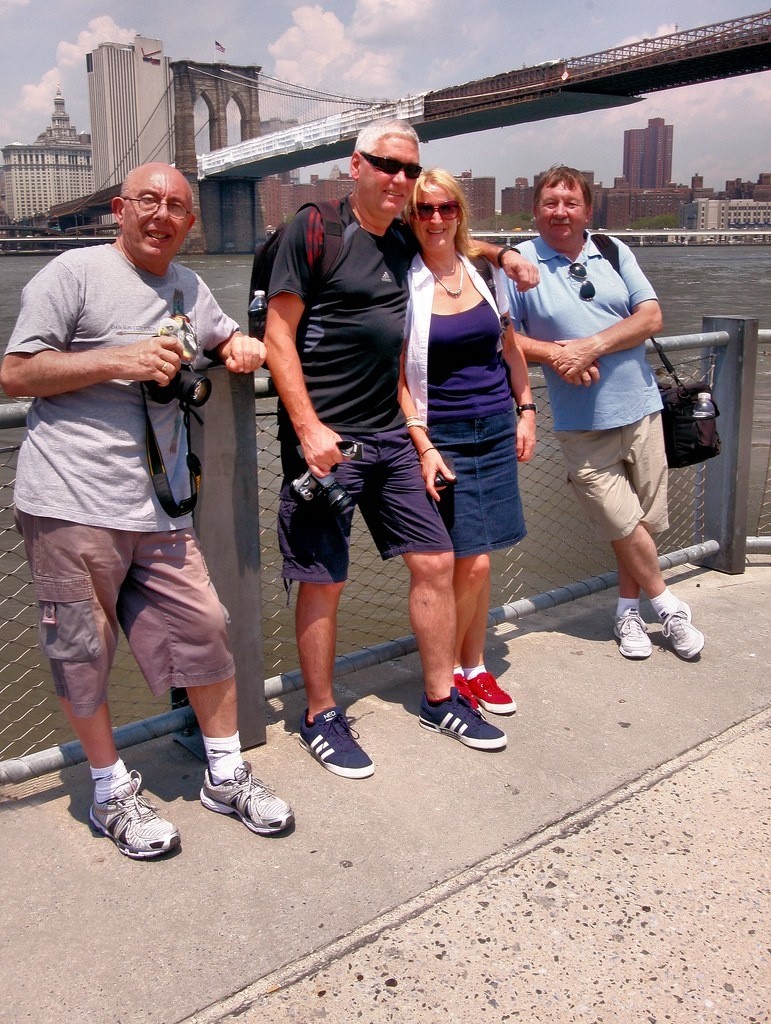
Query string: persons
[498,161,705,659]
[263,117,507,778]
[0,162,295,861]
[397,167,537,714]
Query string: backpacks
[246,204,408,370]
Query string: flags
[215,41,226,53]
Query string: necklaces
[431,258,463,296]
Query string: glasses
[124,195,190,220]
[569,263,596,302]
[410,199,461,221]
[359,150,422,179]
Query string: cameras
[144,359,212,407]
[290,466,354,515]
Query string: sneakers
[613,605,653,659]
[89,769,180,859]
[452,673,483,714]
[420,685,508,749]
[466,671,516,714]
[298,704,374,778]
[658,601,705,659]
[200,759,295,835]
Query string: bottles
[693,392,715,418]
[249,290,267,312]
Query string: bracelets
[407,416,420,419]
[419,447,435,458]
[406,421,427,433]
[516,402,538,416]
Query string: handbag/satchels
[656,379,720,469]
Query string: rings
[160,362,168,373]
